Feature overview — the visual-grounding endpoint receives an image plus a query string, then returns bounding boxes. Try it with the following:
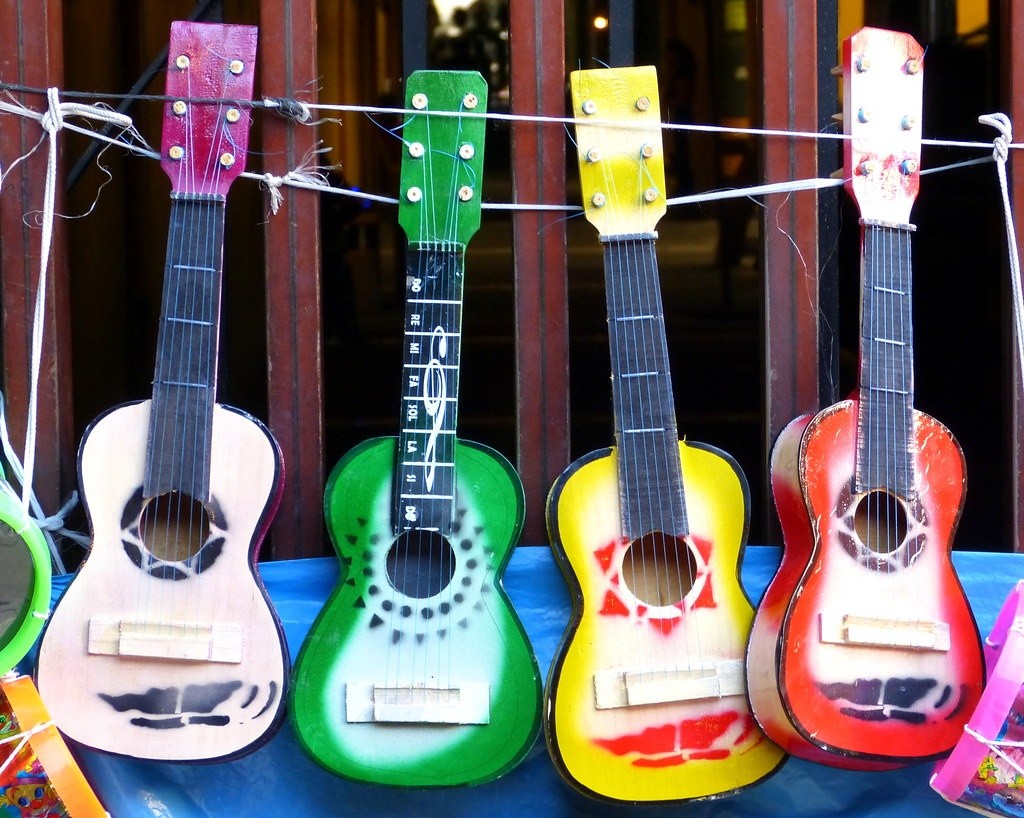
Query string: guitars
[741,26,988,776]
[540,62,791,811]
[288,67,547,794]
[28,16,295,766]
